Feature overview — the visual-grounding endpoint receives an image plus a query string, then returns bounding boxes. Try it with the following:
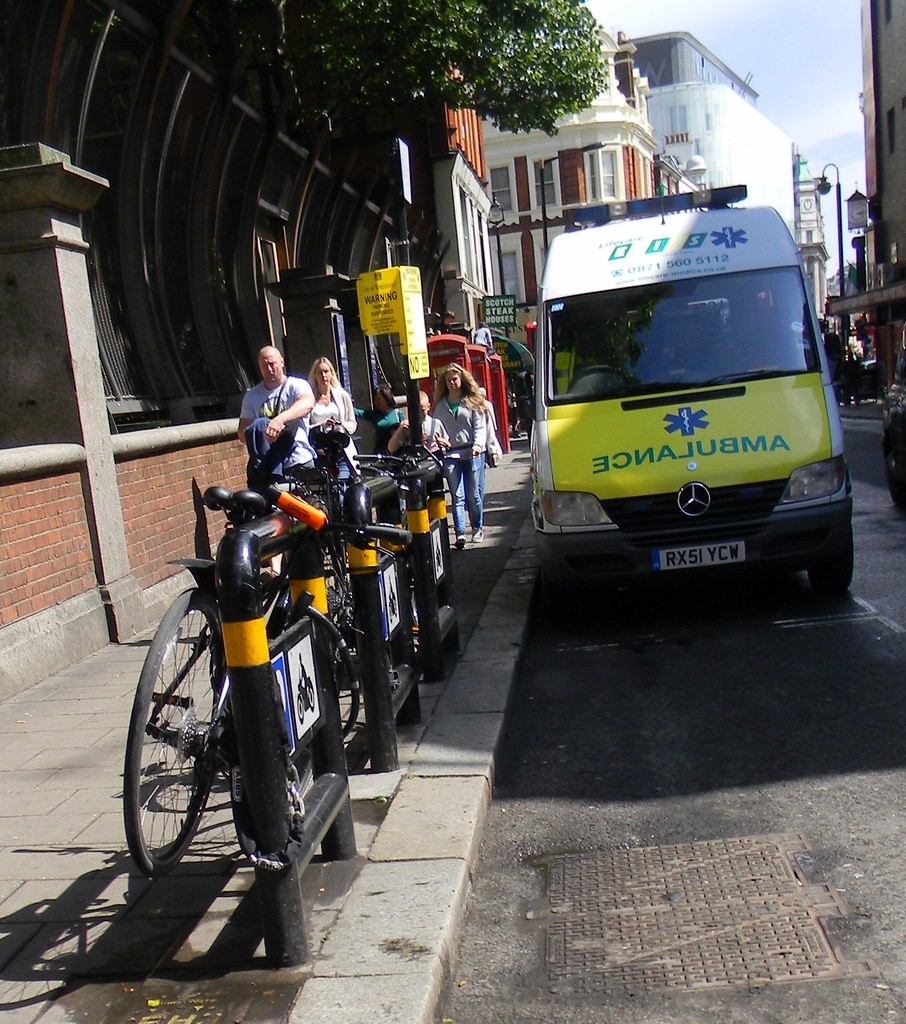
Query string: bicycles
[118,430,474,880]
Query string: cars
[879,317,906,505]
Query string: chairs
[734,306,789,371]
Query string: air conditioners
[869,265,877,290]
[877,263,894,287]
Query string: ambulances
[522,182,856,636]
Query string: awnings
[824,282,906,316]
[489,332,536,375]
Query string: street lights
[488,196,510,339]
[816,163,853,407]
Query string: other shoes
[487,356,493,363]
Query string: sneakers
[471,528,484,543]
[455,535,467,549]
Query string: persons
[237,345,361,513]
[354,361,504,551]
[844,352,864,406]
[504,369,536,450]
[432,309,456,336]
[472,321,498,363]
